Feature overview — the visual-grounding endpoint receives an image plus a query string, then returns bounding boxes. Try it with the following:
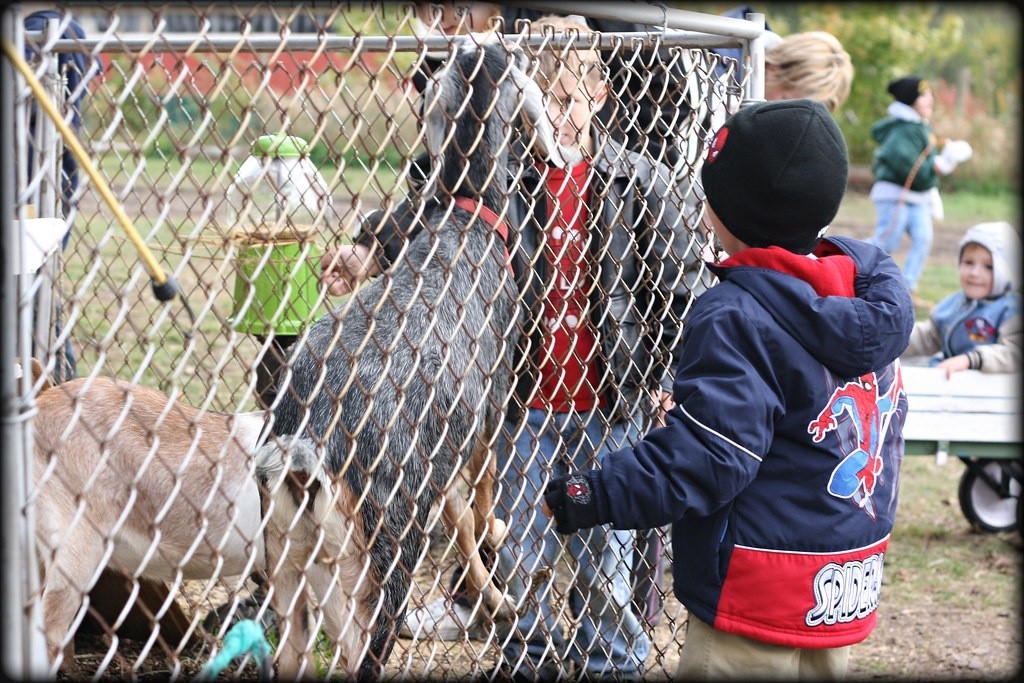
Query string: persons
[547,100,907,679]
[900,220,1020,380]
[860,75,942,311]
[21,0,855,682]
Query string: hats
[700,98,849,248]
[887,75,929,106]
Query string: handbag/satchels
[932,138,973,175]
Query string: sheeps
[16,30,576,683]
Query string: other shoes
[911,298,934,308]
[201,580,280,637]
[475,663,533,683]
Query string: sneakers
[399,598,487,641]
